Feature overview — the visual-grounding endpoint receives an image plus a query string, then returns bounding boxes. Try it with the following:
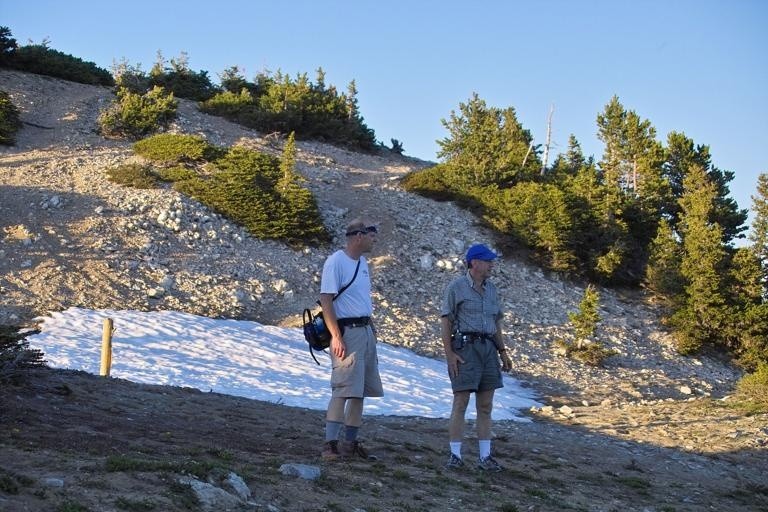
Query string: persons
[440,245,513,473]
[321,219,383,462]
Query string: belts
[462,332,500,350]
[337,318,372,326]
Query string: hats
[466,244,498,263]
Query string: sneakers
[444,452,462,472]
[340,441,377,462]
[322,440,342,462]
[476,454,506,472]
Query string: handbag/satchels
[301,308,333,351]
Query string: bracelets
[499,348,506,354]
